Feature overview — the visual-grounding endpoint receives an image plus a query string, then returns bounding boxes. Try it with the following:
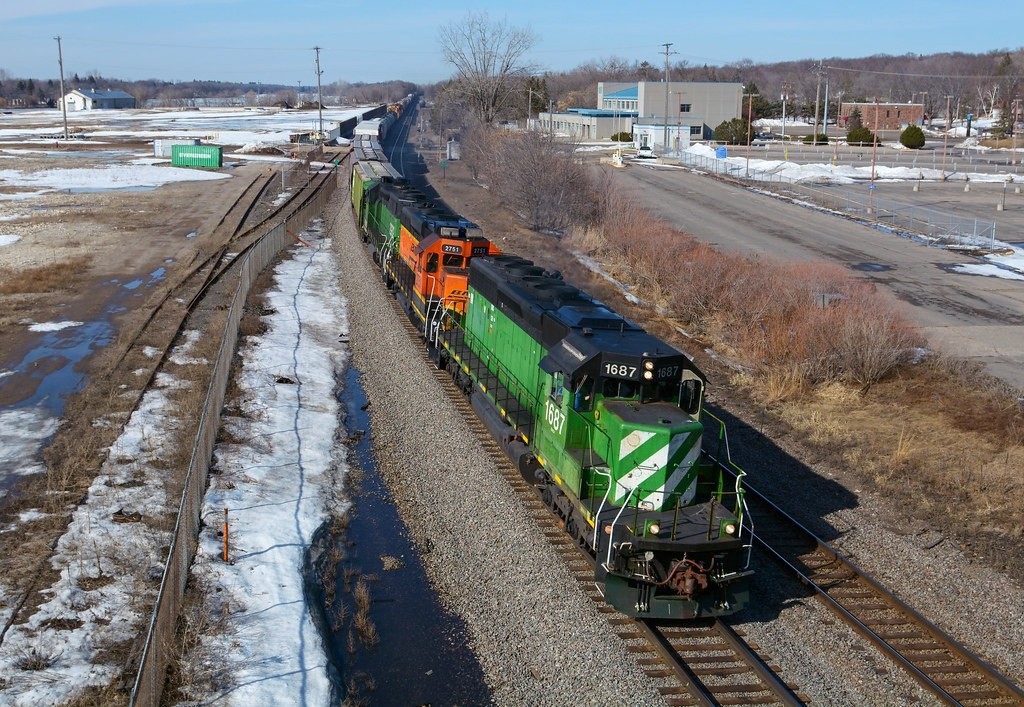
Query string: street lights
[744,92,760,178]
[1012,99,1022,165]
[315,71,324,135]
[297,81,301,98]
[674,91,687,149]
[866,97,890,214]
[781,92,789,138]
[53,36,68,140]
[940,95,954,179]
[920,92,927,129]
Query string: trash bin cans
[716,146,726,158]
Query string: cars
[1017,122,1024,127]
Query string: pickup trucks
[636,146,653,157]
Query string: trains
[348,92,754,619]
